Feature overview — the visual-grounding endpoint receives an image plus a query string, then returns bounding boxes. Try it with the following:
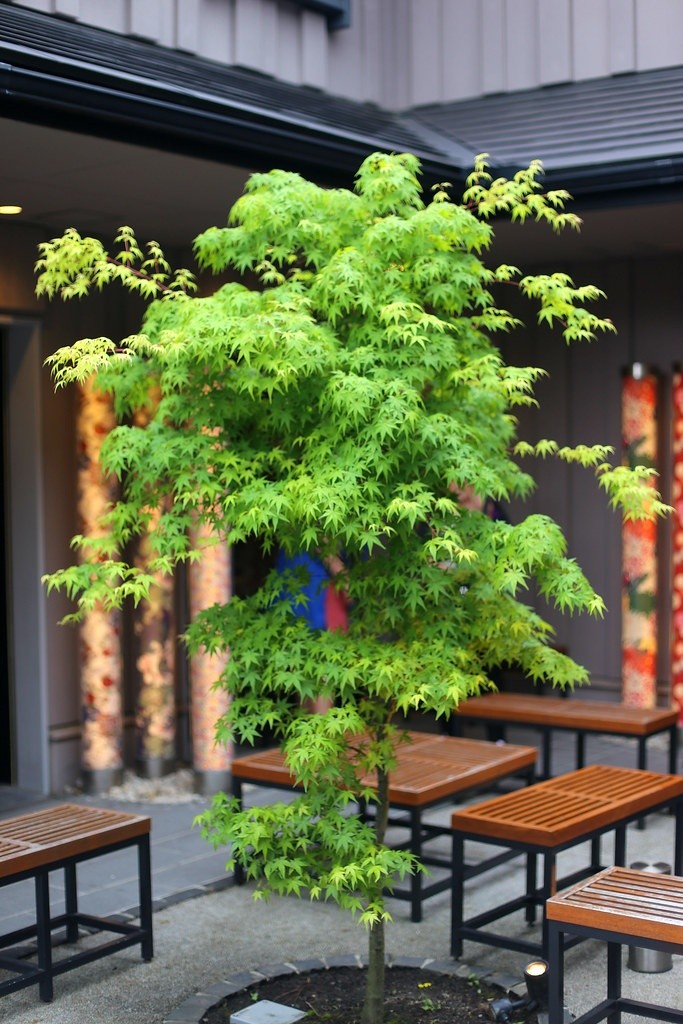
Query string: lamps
[488,959,550,1024]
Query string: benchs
[446,691,679,787]
[451,764,682,964]
[0,804,153,1004]
[545,867,683,1024]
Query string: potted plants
[37,149,673,1024]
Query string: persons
[426,473,525,746]
[274,535,355,717]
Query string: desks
[232,727,539,922]
